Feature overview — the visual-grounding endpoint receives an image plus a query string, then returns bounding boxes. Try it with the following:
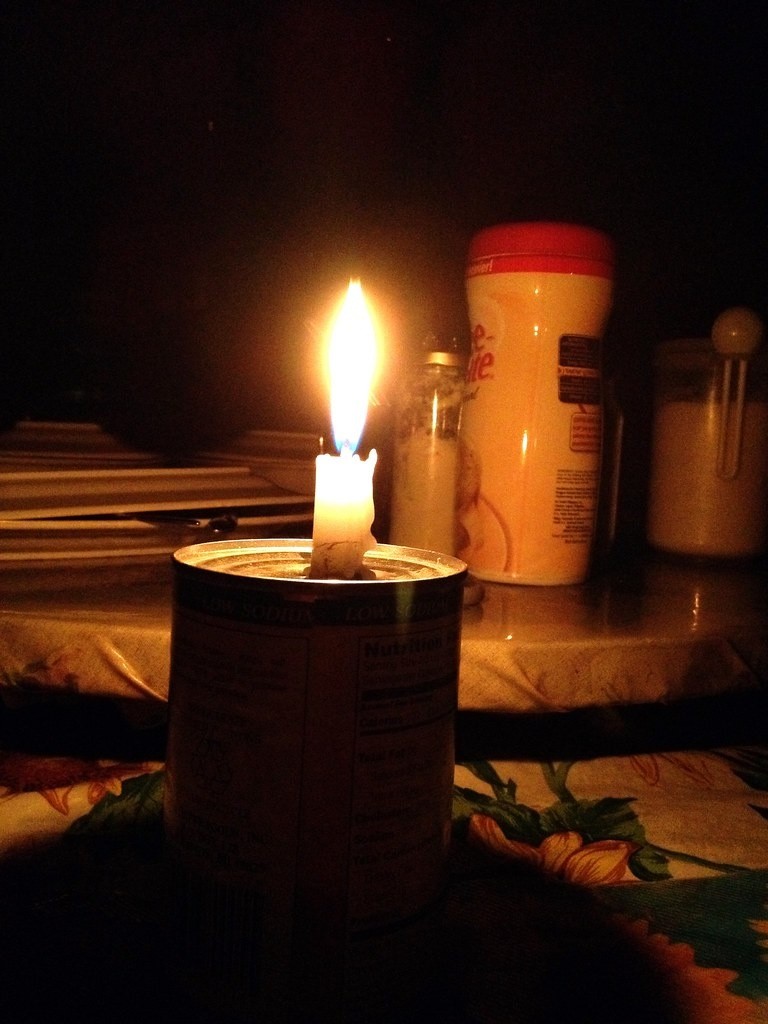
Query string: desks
[0,515,768,1024]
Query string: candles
[311,277,377,579]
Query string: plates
[191,429,323,463]
[0,421,159,459]
[0,463,319,589]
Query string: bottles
[648,308,767,556]
[390,351,469,558]
[459,222,613,586]
